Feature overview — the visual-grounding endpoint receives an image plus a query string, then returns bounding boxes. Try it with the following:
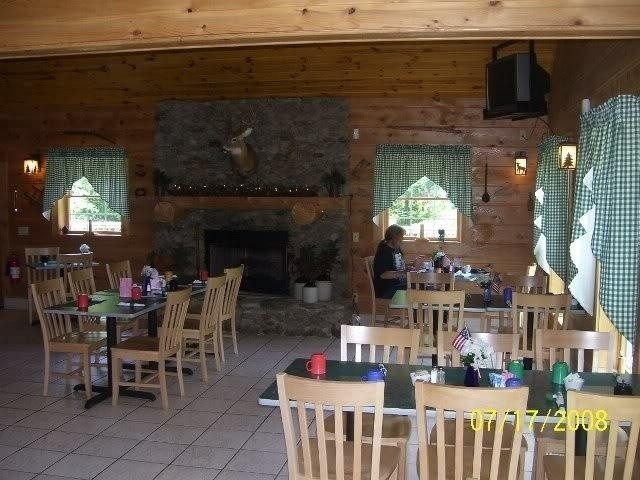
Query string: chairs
[502,274,548,297]
[543,391,640,480]
[406,288,464,354]
[406,271,454,292]
[534,328,630,454]
[363,256,405,326]
[24,247,244,411]
[276,374,406,480]
[430,329,529,452]
[511,292,570,369]
[414,379,531,480]
[316,326,422,448]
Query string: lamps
[557,142,578,173]
[23,153,41,176]
[514,152,528,176]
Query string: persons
[373,225,449,327]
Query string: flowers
[460,337,493,364]
[432,254,449,261]
[476,271,496,287]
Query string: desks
[389,290,588,370]
[454,275,545,295]
[257,357,619,415]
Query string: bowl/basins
[564,380,584,391]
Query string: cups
[78,294,89,309]
[505,360,525,388]
[360,367,384,382]
[306,354,327,375]
[132,286,142,301]
[552,361,568,385]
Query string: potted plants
[321,168,344,198]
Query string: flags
[452,327,471,353]
[492,276,500,293]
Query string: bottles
[146,283,152,293]
[614,383,633,395]
[504,287,512,308]
[431,365,446,385]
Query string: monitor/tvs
[485,53,551,109]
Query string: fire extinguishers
[8,251,21,284]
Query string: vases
[482,288,491,301]
[464,365,480,387]
[434,262,441,274]
